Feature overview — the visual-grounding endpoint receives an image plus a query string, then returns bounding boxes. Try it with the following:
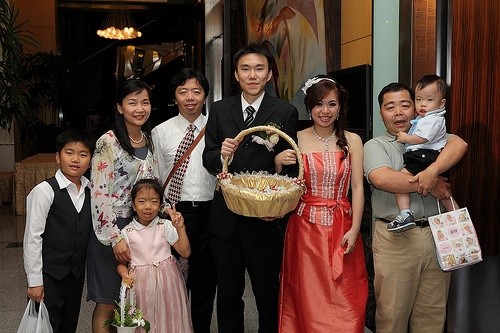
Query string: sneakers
[386,210,417,234]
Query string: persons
[275,75,368,333]
[363,75,468,333]
[202,43,299,333]
[151,69,218,333]
[87,79,171,333]
[117,179,194,333]
[23,130,94,333]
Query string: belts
[175,201,211,209]
[378,218,429,227]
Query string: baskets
[216,125,306,218]
[112,285,138,333]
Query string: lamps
[97,0,142,40]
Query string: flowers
[260,122,283,145]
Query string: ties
[166,125,197,206]
[244,106,255,129]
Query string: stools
[0,171,14,204]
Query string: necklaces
[129,132,144,144]
[312,125,336,151]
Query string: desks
[14,153,59,216]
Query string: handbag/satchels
[428,196,483,272]
[16,298,53,333]
